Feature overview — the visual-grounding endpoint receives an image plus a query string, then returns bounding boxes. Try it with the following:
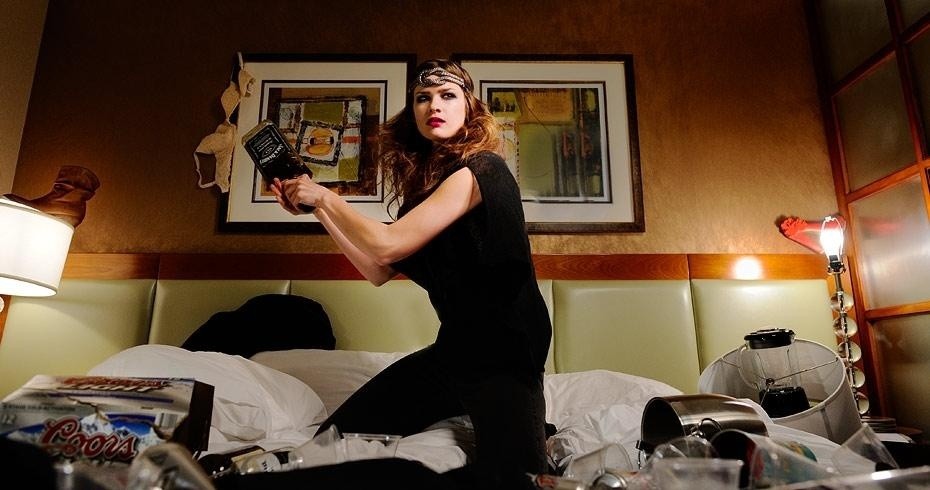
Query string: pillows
[252,348,420,423]
[86,349,327,445]
[545,369,688,428]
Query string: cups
[288,422,405,469]
[562,430,842,490]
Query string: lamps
[0,194,76,299]
[820,216,898,434]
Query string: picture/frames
[450,49,649,238]
[214,52,416,239]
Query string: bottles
[240,118,317,214]
[193,446,292,479]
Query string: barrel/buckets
[633,393,775,490]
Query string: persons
[269,56,557,487]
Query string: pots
[636,394,770,471]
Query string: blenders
[737,326,811,420]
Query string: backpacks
[180,293,336,358]
